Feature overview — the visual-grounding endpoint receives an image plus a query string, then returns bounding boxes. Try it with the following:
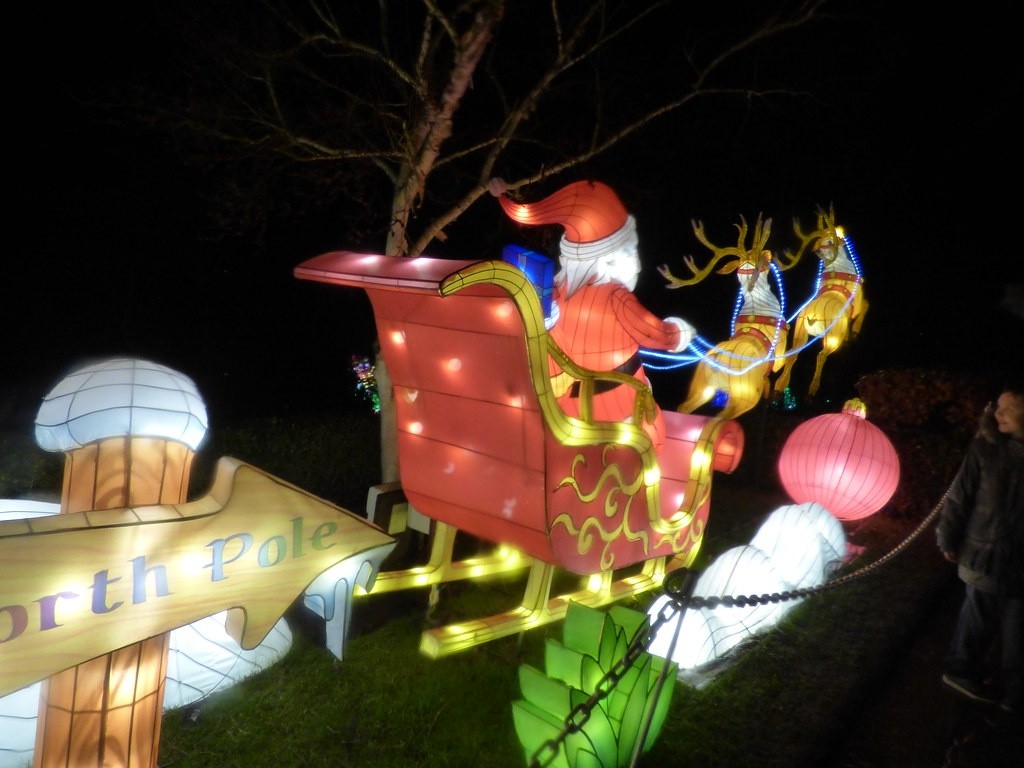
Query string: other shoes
[1000,690,1023,716]
[942,668,996,707]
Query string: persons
[936,386,1024,713]
[488,178,696,466]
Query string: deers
[657,200,870,415]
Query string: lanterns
[778,398,902,524]
